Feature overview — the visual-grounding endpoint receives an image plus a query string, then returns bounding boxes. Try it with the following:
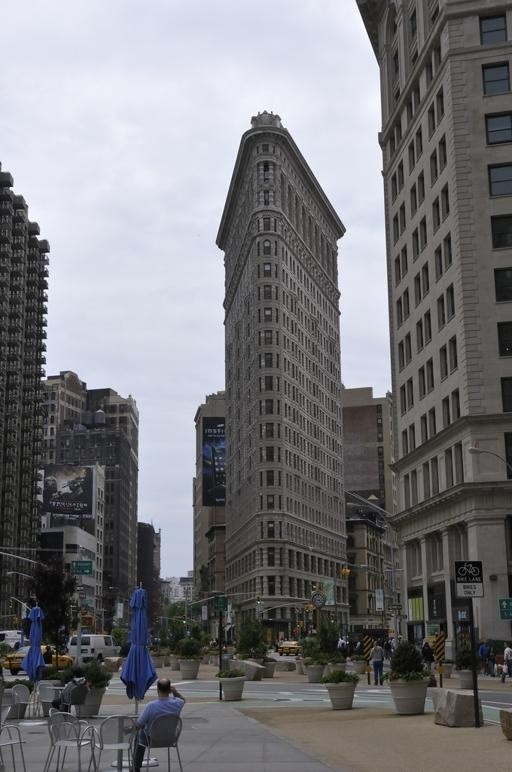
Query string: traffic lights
[256,595,262,605]
[182,615,186,624]
[8,599,13,609]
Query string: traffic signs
[70,559,93,575]
[498,598,512,621]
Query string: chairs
[0,680,183,772]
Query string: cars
[277,639,303,656]
[0,629,30,651]
[3,645,72,676]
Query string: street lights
[347,502,399,655]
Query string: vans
[64,634,122,665]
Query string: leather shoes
[124,726,133,734]
[130,767,140,772]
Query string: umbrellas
[119,582,158,721]
[20,601,49,720]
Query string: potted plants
[150,614,277,701]
[379,637,434,715]
[292,616,369,710]
[453,645,483,689]
[117,641,131,668]
[0,655,113,717]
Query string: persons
[129,678,186,772]
[368,633,512,689]
